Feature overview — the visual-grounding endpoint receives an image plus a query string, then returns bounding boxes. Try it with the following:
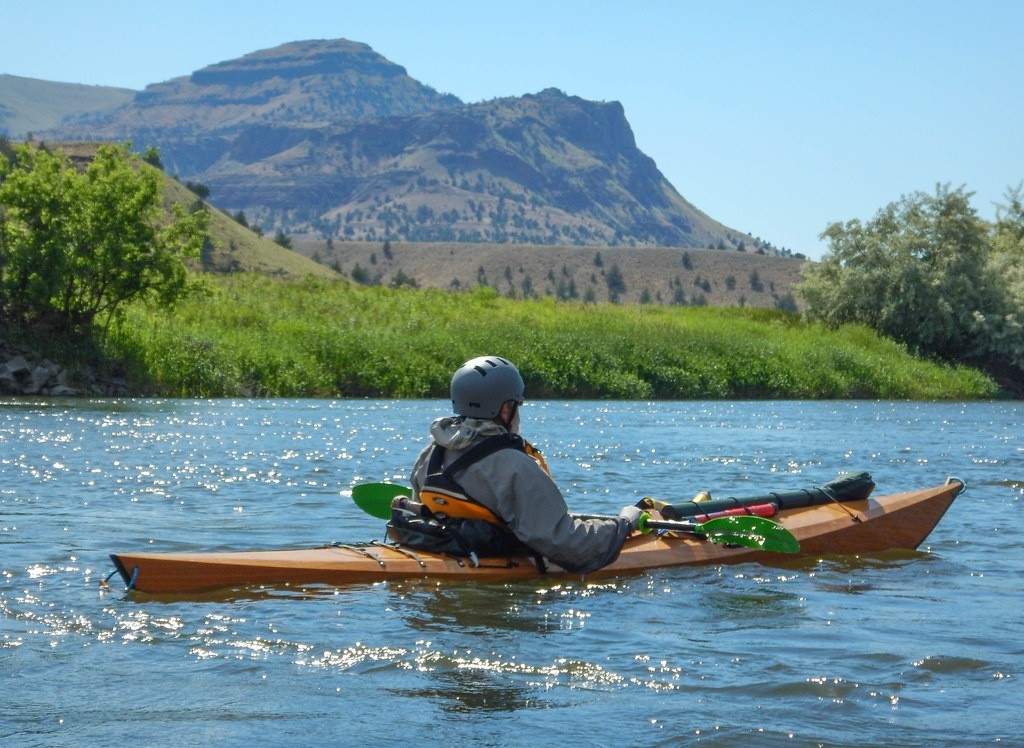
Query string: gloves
[618,505,644,536]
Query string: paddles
[350,482,804,555]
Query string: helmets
[450,356,525,419]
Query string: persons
[410,356,645,575]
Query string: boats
[108,474,970,595]
